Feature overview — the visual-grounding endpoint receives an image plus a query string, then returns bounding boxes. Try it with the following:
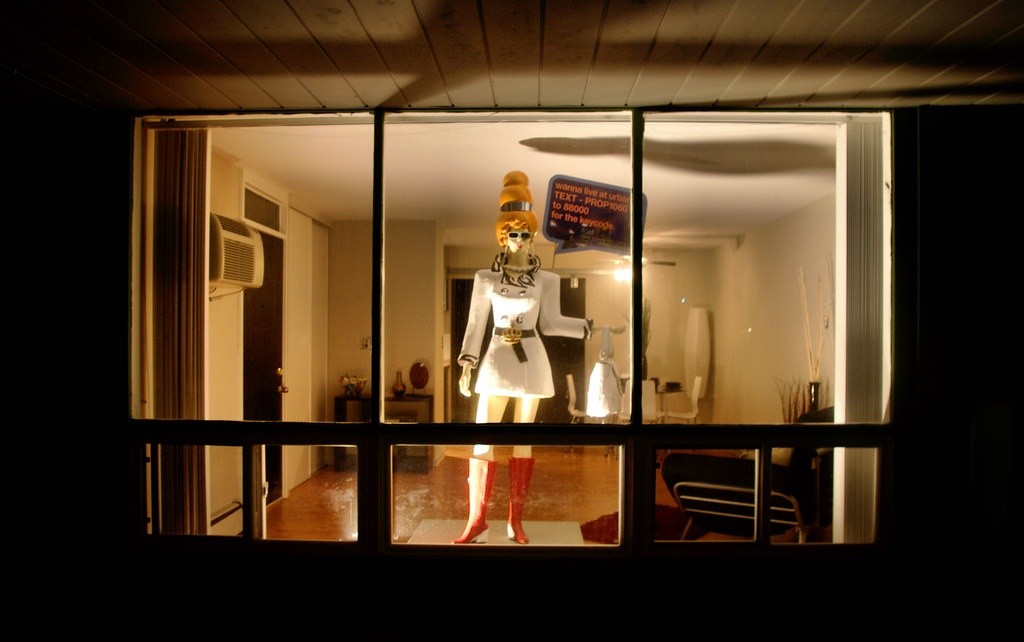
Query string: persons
[451,171,627,544]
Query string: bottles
[392,371,405,396]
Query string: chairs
[662,407,835,542]
[666,376,702,424]
[566,375,608,457]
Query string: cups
[651,378,658,391]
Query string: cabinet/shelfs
[335,393,435,471]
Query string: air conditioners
[209,214,264,288]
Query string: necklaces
[504,265,533,273]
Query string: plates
[410,362,428,389]
[667,382,681,386]
